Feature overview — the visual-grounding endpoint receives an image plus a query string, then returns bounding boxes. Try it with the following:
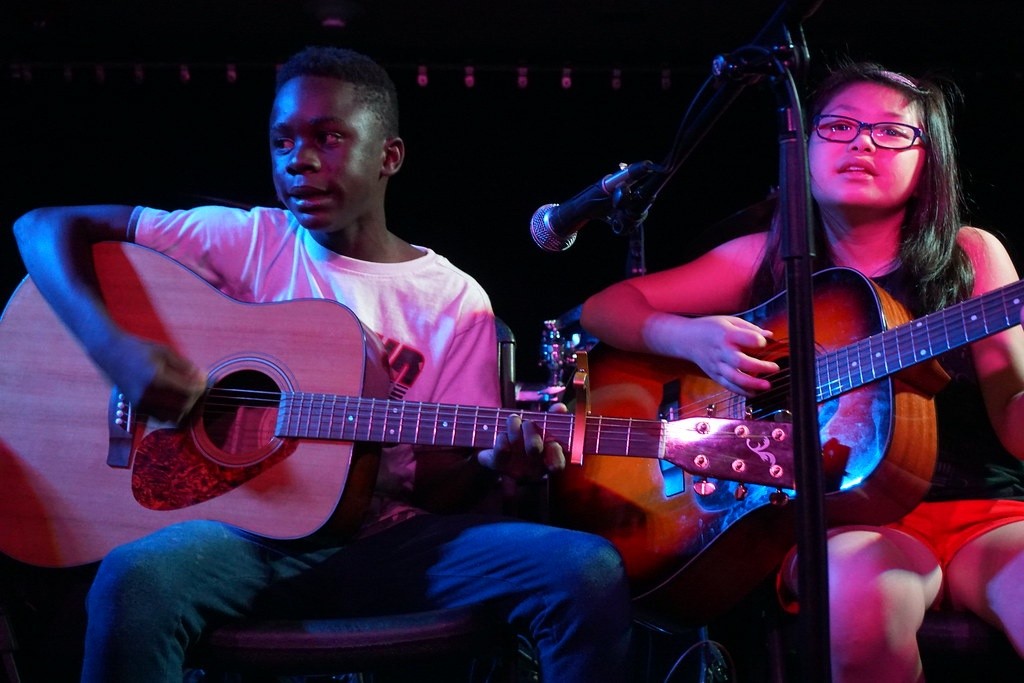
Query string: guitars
[513,263,1024,628]
[0,237,851,569]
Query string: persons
[12,49,634,683]
[579,62,1024,683]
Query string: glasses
[812,114,931,154]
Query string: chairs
[192,602,484,683]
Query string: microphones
[531,161,653,252]
